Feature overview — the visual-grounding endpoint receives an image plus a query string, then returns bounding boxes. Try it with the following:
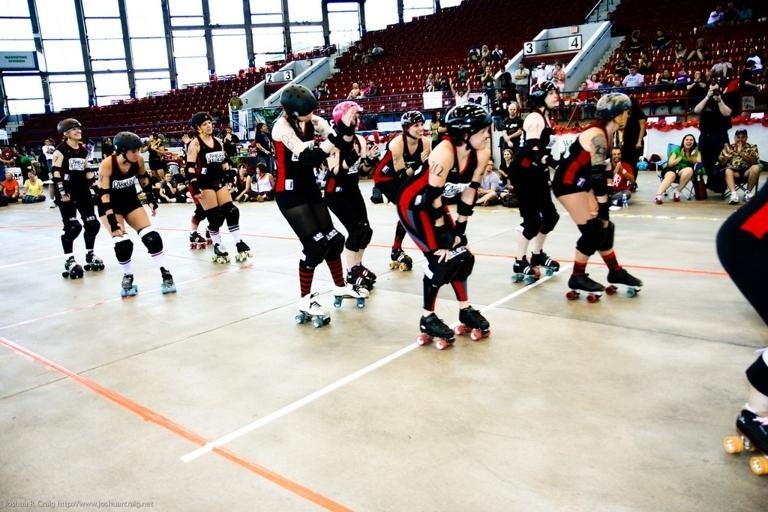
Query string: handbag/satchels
[693,174,708,200]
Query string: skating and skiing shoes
[60,250,105,280]
[160,266,176,295]
[512,251,562,285]
[565,269,644,304]
[189,227,253,265]
[388,245,415,272]
[120,271,138,297]
[331,261,377,310]
[723,403,768,476]
[295,293,331,328]
[417,305,491,350]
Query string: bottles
[621,194,629,210]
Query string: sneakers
[743,189,753,202]
[728,191,740,204]
[653,187,683,205]
[49,199,57,208]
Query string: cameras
[711,87,721,97]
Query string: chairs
[132,77,229,148]
[228,43,337,148]
[308,1,563,118]
[561,1,768,129]
[11,99,131,155]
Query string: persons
[716,179,768,457]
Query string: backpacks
[648,154,663,171]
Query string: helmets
[333,100,363,130]
[111,131,145,154]
[444,102,493,141]
[280,83,319,120]
[191,113,215,129]
[527,81,556,107]
[56,117,83,134]
[596,91,634,118]
[398,110,426,129]
